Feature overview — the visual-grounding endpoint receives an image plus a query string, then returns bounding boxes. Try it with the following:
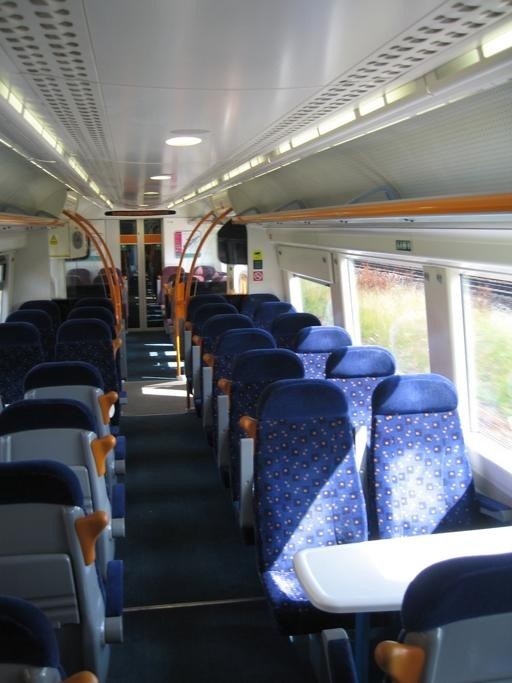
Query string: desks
[292,525,512,613]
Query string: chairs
[368,372,481,539]
[369,551,512,683]
[227,346,308,532]
[256,376,371,637]
[324,345,397,476]
